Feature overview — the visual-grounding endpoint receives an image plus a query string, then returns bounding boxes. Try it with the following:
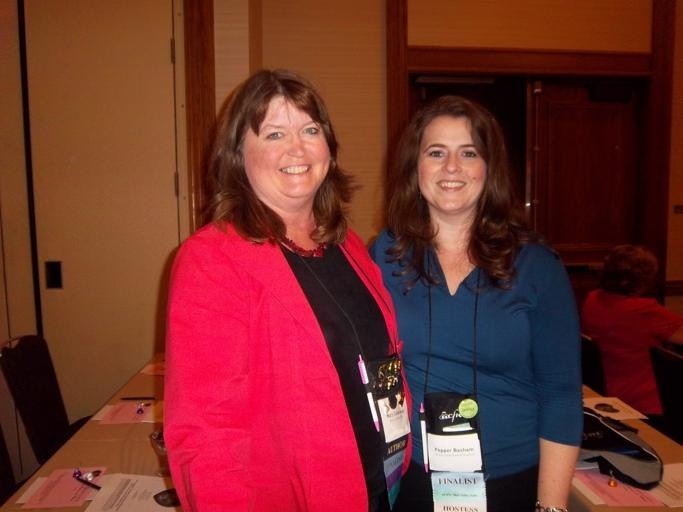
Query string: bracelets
[533,499,569,512]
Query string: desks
[0,352,683,512]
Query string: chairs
[0,335,94,465]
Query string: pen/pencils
[419,402,429,473]
[120,397,155,399]
[74,475,101,490]
[358,354,380,433]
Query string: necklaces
[275,225,329,258]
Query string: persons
[580,241,683,437]
[161,70,416,510]
[365,94,585,511]
